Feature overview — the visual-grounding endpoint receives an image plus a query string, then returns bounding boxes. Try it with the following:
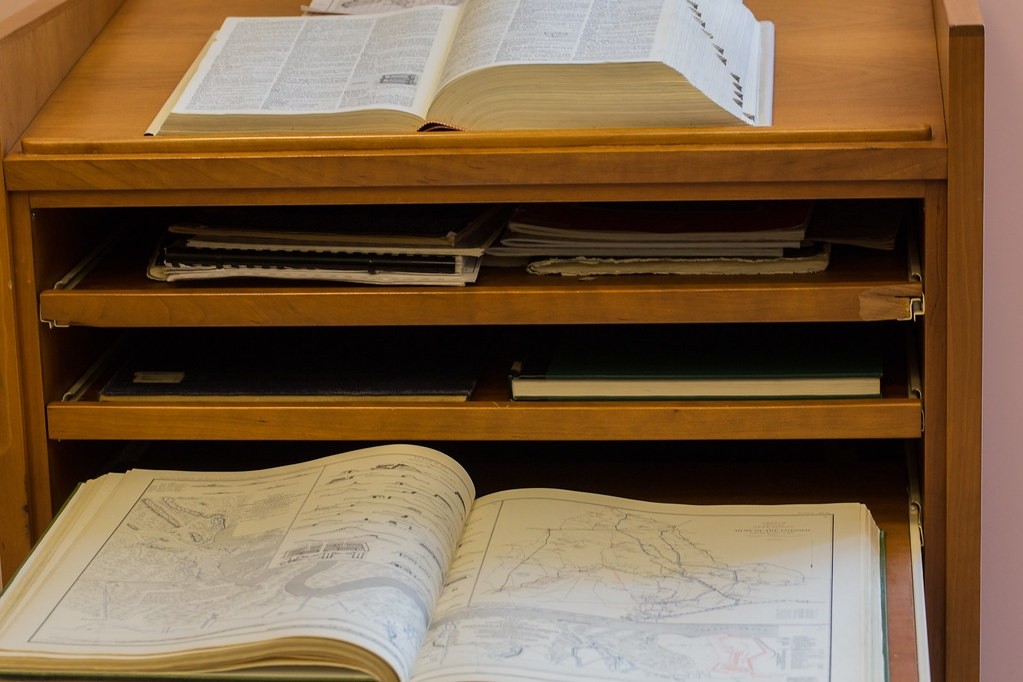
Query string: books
[93,325,895,402]
[145,200,897,287]
[142,0,775,136]
[0,444,890,682]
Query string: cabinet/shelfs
[0,0,987,682]
